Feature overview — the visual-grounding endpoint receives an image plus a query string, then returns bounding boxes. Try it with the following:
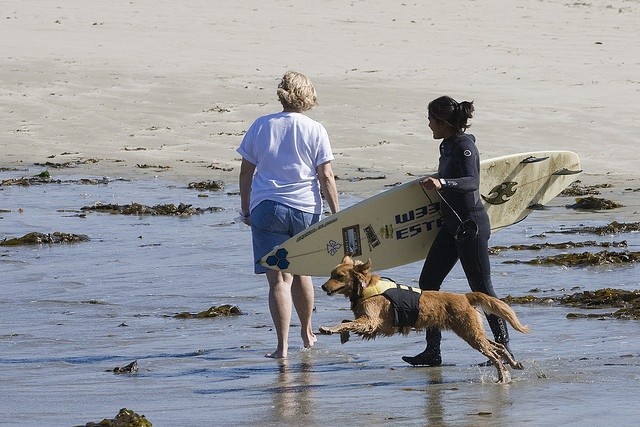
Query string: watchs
[439,177,447,184]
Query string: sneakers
[402,348,441,367]
[478,342,515,367]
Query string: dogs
[318,250,529,384]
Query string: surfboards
[256,151,582,277]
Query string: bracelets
[239,211,250,219]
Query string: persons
[235,72,340,359]
[402,96,515,367]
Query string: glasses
[440,139,453,156]
